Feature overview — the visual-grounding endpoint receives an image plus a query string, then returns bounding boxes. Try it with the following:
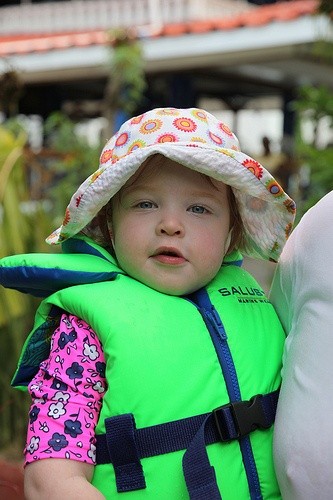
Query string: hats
[44,107,297,265]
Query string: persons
[264,188,333,500]
[0,105,296,500]
